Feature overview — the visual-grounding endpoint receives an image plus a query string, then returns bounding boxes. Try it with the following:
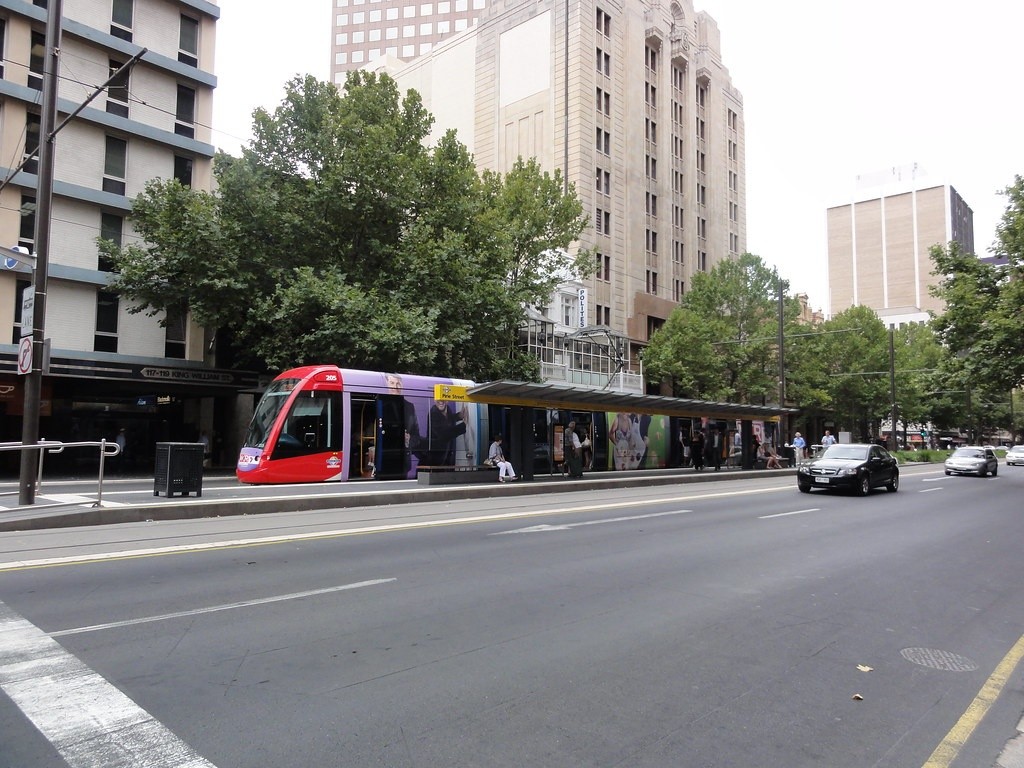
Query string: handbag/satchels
[484,458,497,467]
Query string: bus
[233,365,785,488]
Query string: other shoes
[498,478,506,483]
[510,477,518,482]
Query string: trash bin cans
[154,442,205,498]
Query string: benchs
[416,464,500,484]
[754,456,790,469]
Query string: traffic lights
[920,429,925,439]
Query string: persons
[489,434,518,483]
[563,421,592,476]
[374,374,470,479]
[682,429,783,469]
[610,412,652,472]
[793,432,806,467]
[821,430,837,449]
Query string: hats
[795,432,802,436]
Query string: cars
[1005,445,1024,466]
[796,443,899,496]
[984,445,1011,450]
[944,446,999,477]
